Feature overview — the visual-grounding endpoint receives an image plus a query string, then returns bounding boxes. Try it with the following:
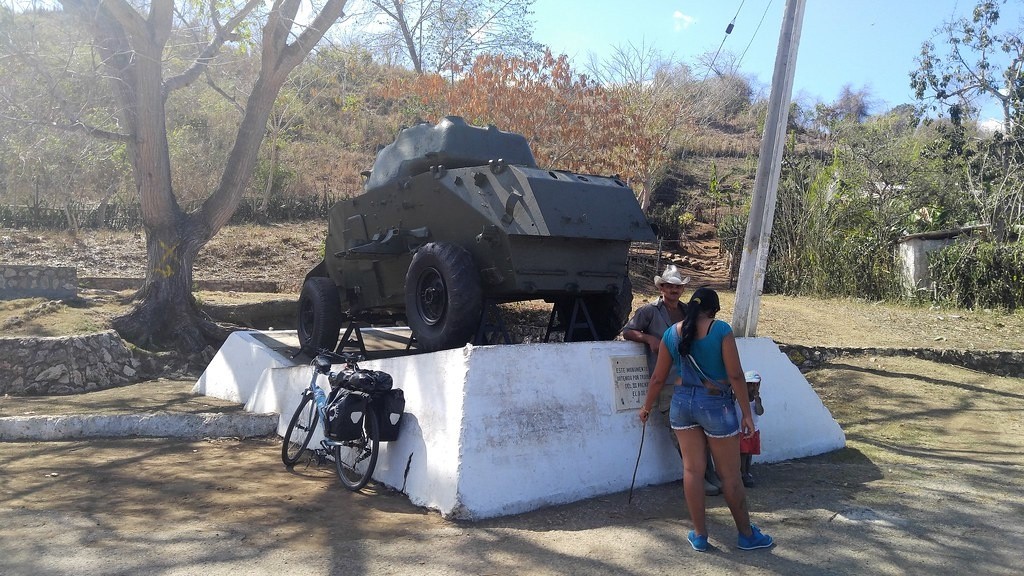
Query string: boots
[678,449,719,496]
[705,451,724,493]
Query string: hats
[654,264,691,289]
[743,371,762,383]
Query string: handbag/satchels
[322,388,371,442]
[364,389,406,442]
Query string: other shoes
[744,473,755,487]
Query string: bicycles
[280,337,382,493]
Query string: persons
[623,264,764,496]
[639,288,773,552]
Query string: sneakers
[737,524,774,550]
[687,529,709,552]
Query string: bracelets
[641,405,649,414]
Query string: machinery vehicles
[292,119,657,355]
